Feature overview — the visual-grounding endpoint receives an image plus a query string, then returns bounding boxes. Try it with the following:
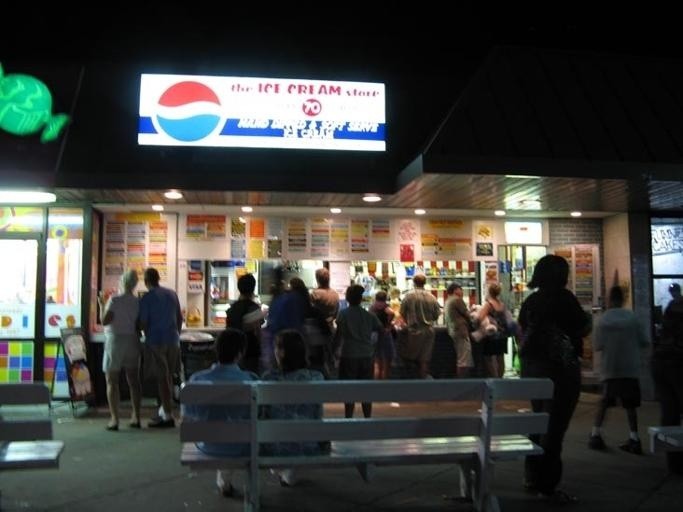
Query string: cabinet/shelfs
[419,272,476,292]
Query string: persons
[182,266,509,498]
[513,255,682,503]
[96,268,182,431]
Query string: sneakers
[148,417,175,428]
[587,434,606,450]
[617,438,642,453]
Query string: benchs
[178,377,555,508]
